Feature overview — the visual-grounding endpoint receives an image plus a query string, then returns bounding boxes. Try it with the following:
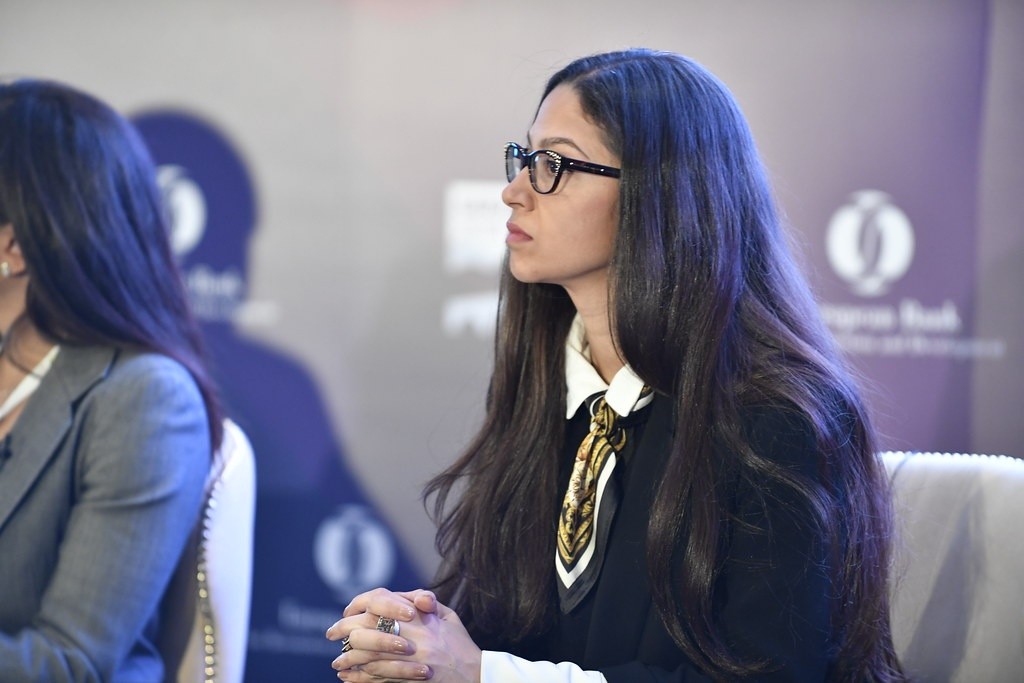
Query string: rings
[341,636,353,653]
[376,616,400,636]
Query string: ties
[551,384,655,614]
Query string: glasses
[504,141,625,195]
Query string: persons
[0,78,226,683]
[326,48,909,683]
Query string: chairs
[162,417,255,683]
[874,451,1023,683]
